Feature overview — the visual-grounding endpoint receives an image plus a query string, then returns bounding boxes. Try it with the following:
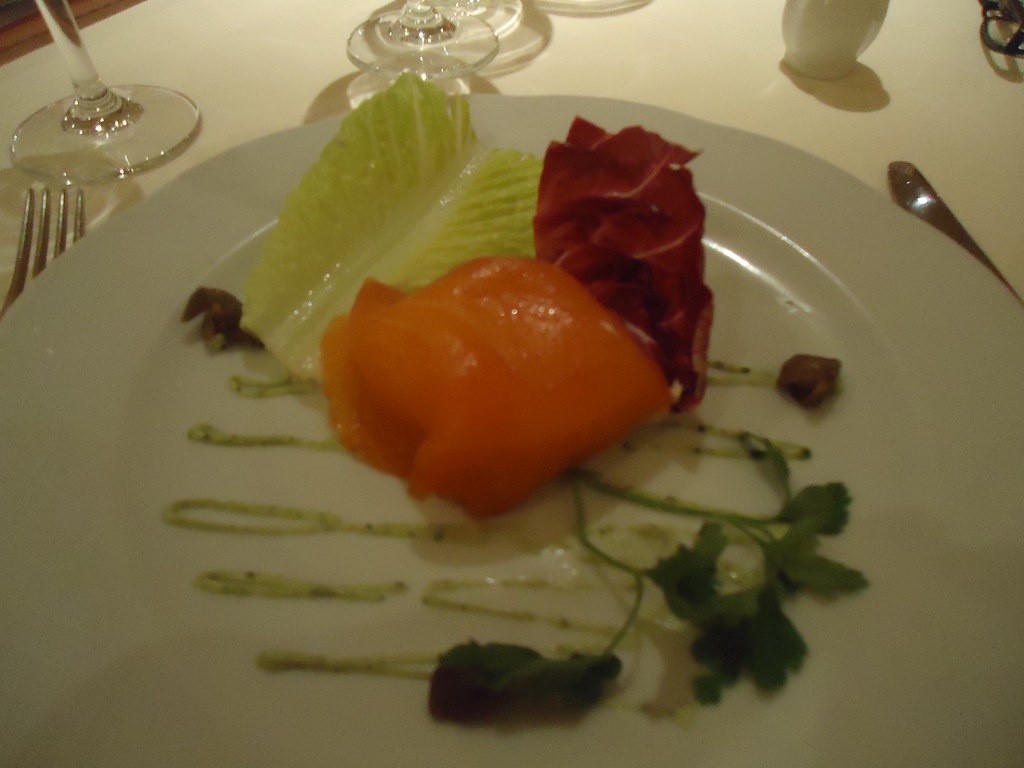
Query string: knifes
[888,160,1021,302]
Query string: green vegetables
[425,432,868,718]
[238,74,544,386]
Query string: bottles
[780,1,890,81]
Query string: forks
[0,187,85,320]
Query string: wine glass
[10,1,202,188]
[347,0,653,83]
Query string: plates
[1,93,1024,768]
